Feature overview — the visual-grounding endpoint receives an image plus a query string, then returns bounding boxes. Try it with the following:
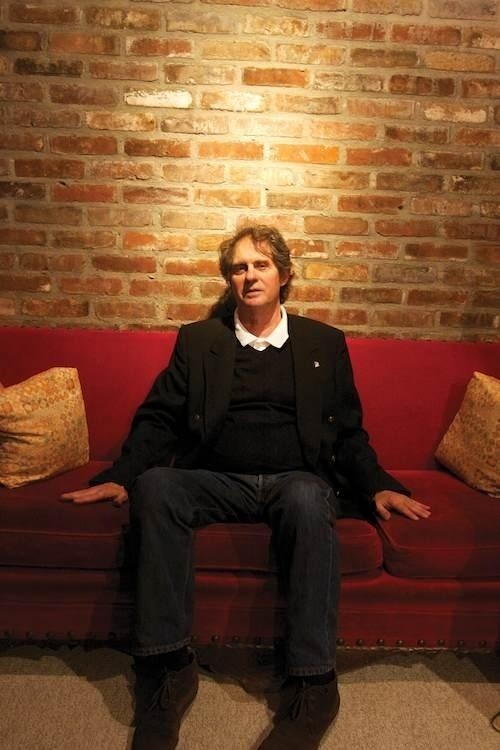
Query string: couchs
[0,327,500,655]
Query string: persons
[59,221,434,748]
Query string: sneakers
[131,652,199,749]
[259,672,343,749]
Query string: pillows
[434,371,500,498]
[0,368,91,490]
